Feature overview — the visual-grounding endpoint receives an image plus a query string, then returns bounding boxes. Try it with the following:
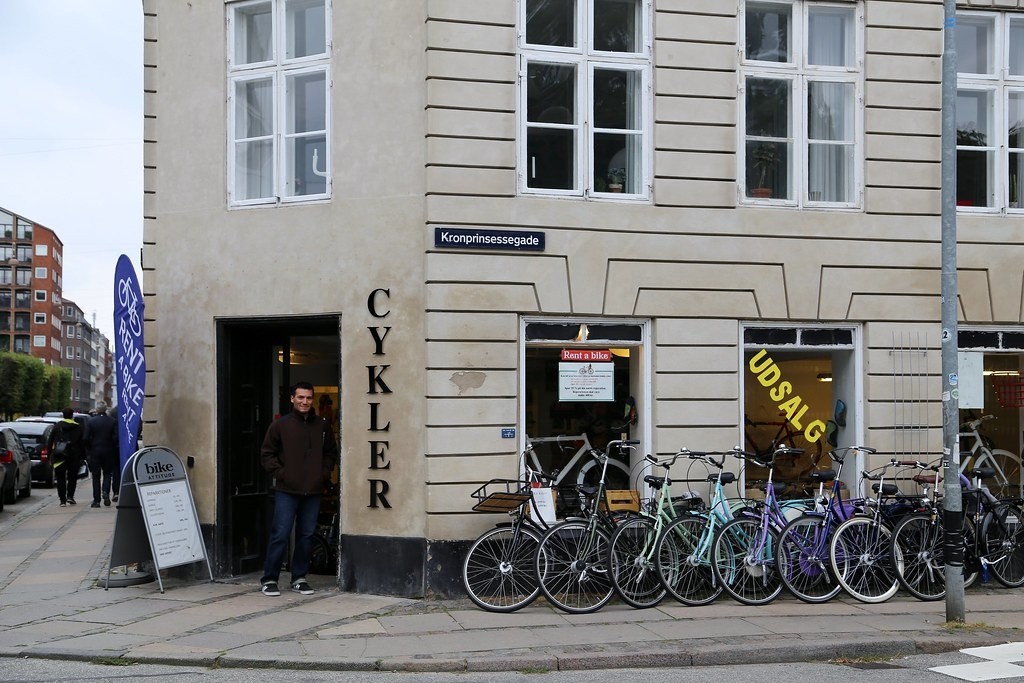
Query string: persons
[0,414,4,423]
[260,381,336,596]
[85,400,120,507]
[47,408,82,507]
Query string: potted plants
[606,168,628,193]
[750,128,783,200]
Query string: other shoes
[61,500,67,506]
[91,502,100,507]
[102,491,111,506]
[66,497,77,504]
[112,493,118,502]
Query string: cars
[0,427,36,512]
[0,410,110,489]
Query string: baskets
[470,478,532,515]
[598,489,640,516]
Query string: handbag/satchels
[52,440,71,462]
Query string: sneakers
[291,580,315,594]
[262,579,280,596]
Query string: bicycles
[233,481,340,575]
[744,409,823,480]
[524,422,632,510]
[959,415,1022,503]
[461,432,1023,606]
